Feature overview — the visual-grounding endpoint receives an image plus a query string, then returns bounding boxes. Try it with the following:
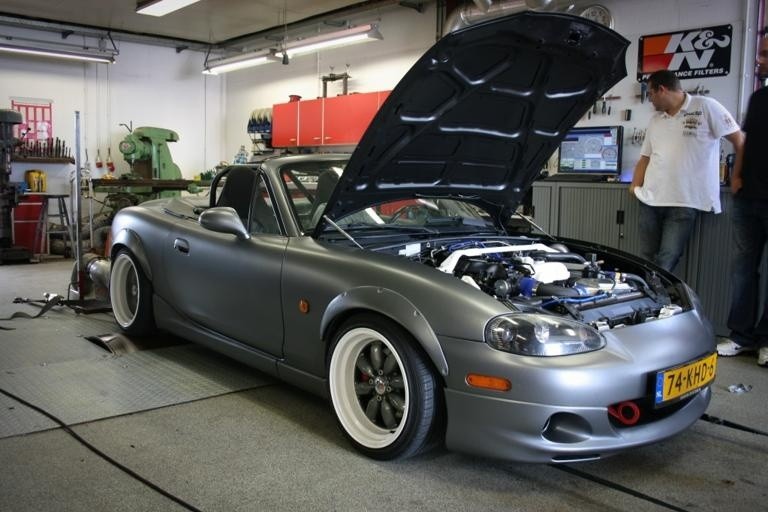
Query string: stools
[35,194,78,261]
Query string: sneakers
[714,338,755,356]
[757,346,768,366]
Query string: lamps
[1,25,119,68]
[196,14,386,75]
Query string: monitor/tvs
[558,124,624,176]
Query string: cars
[109,9,718,461]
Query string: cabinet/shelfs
[271,98,323,148]
[11,191,52,257]
[531,183,733,339]
[320,92,378,147]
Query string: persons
[716,26,768,368]
[629,68,746,273]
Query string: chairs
[216,166,280,235]
[307,165,385,230]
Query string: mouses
[607,178,617,182]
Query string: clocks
[577,4,615,32]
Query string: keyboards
[544,174,604,182]
